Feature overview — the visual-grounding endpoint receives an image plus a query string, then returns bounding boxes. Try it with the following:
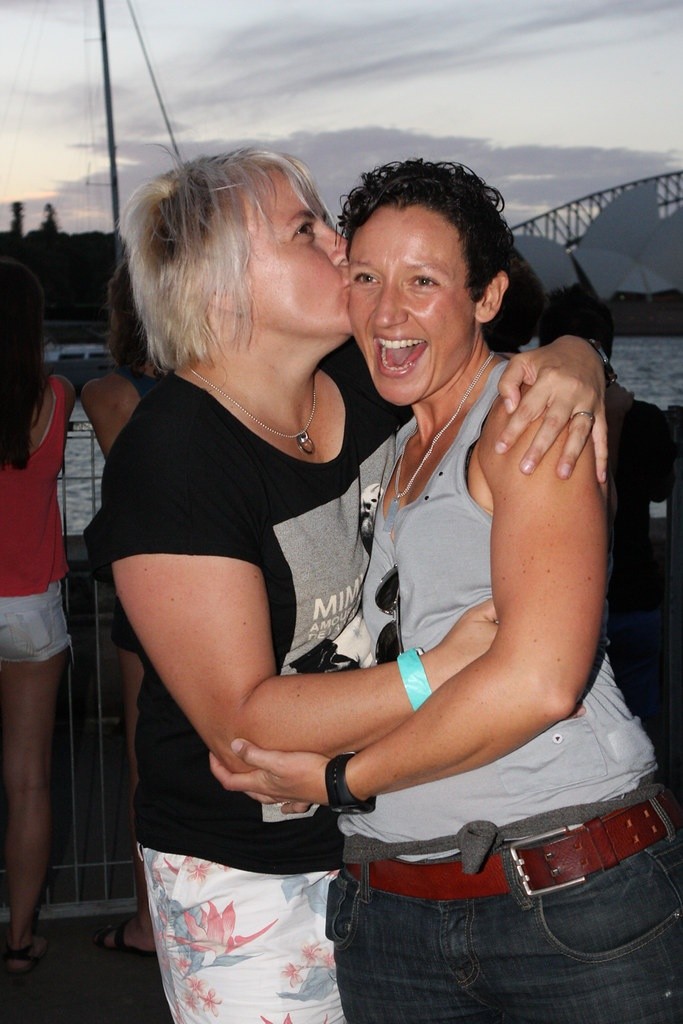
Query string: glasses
[367,560,403,664]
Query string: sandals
[3,934,49,974]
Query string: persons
[488,254,674,719]
[0,260,76,974]
[83,261,162,956]
[207,159,683,1024]
[101,149,608,1024]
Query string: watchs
[326,751,376,814]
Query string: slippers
[93,916,158,956]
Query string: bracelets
[585,337,617,384]
[397,647,431,710]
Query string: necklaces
[382,352,494,533]
[188,366,316,453]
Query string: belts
[346,796,674,902]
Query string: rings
[571,411,595,424]
